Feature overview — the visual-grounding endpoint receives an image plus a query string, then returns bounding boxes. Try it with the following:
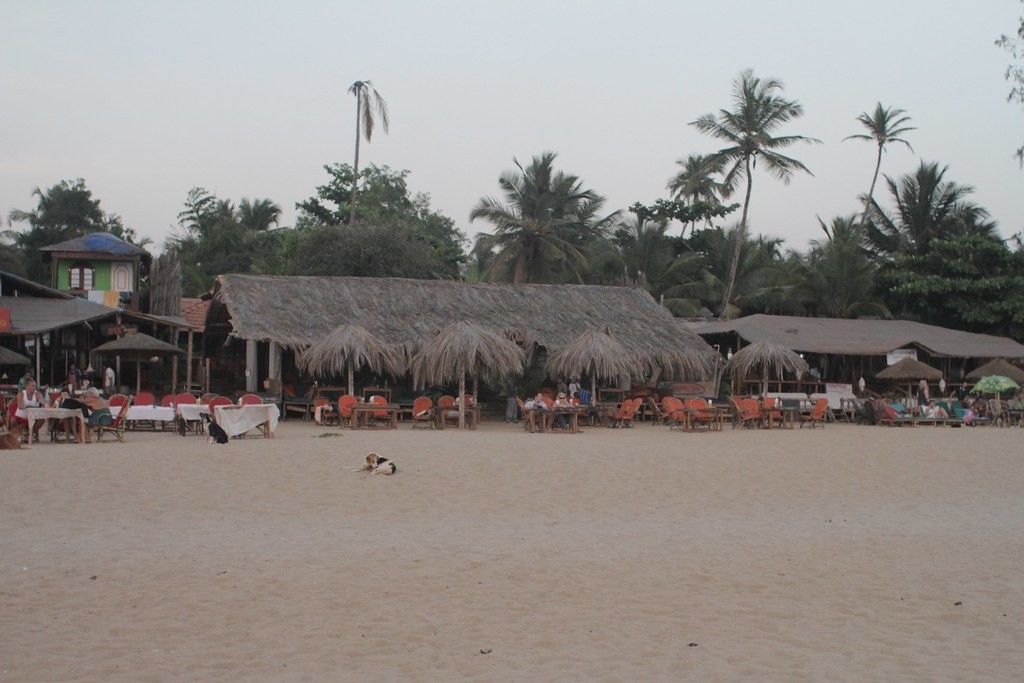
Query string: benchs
[766,393,816,415]
[810,393,862,410]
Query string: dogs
[198,412,229,446]
[343,453,396,476]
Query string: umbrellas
[966,358,1024,381]
[544,327,642,408]
[970,375,1021,400]
[0,346,31,365]
[875,358,943,399]
[298,323,404,396]
[91,332,188,396]
[408,320,527,427]
[725,337,810,397]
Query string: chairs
[281,376,479,430]
[0,385,266,442]
[516,393,1024,431]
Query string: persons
[17,362,115,443]
[924,382,1024,428]
[525,375,592,433]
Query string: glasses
[558,397,566,400]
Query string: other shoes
[530,429,535,433]
[86,438,91,444]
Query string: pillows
[283,384,296,398]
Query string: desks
[597,389,625,403]
[433,406,482,430]
[677,408,727,431]
[27,408,88,443]
[214,403,280,439]
[109,405,175,432]
[359,387,391,404]
[178,404,211,435]
[317,387,346,398]
[539,406,588,432]
[346,404,400,430]
[758,407,798,429]
[1006,409,1024,428]
